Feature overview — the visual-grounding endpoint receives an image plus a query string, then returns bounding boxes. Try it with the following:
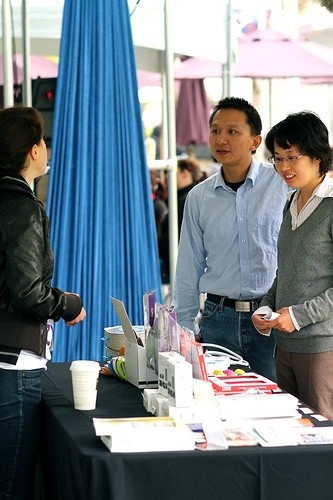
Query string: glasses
[267,154,304,164]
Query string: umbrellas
[0,31,333,155]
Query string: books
[93,417,258,453]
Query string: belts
[207,292,263,313]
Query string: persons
[0,108,86,500]
[174,98,289,385]
[158,159,207,283]
[251,113,333,420]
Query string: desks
[34,361,333,500]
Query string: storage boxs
[109,294,193,408]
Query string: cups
[70,359,101,410]
